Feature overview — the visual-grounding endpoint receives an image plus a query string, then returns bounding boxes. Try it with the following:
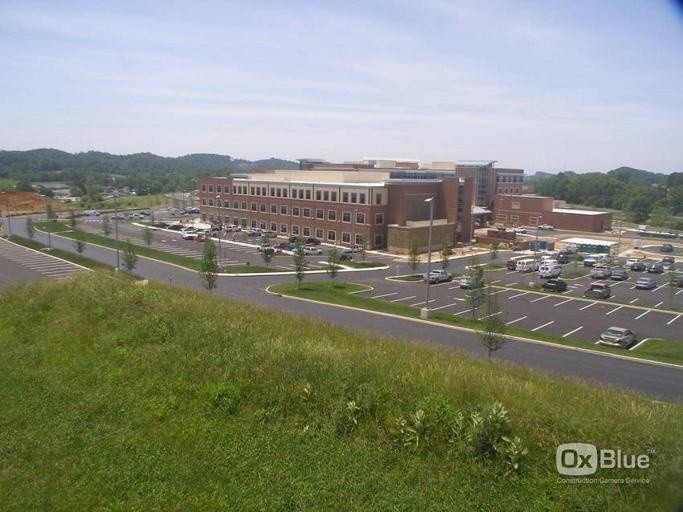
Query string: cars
[599,326,638,349]
[506,246,682,298]
[660,243,673,252]
[344,244,363,253]
[460,276,485,289]
[152,221,242,241]
[257,235,325,257]
[166,205,201,217]
[336,253,353,262]
[655,230,683,239]
[496,222,507,231]
[110,210,151,219]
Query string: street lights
[183,189,189,210]
[420,195,438,320]
[215,194,224,273]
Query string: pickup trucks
[538,223,554,231]
[511,226,527,234]
[423,269,453,284]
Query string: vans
[259,230,277,238]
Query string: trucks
[84,210,100,217]
[638,225,647,233]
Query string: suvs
[248,227,264,237]
